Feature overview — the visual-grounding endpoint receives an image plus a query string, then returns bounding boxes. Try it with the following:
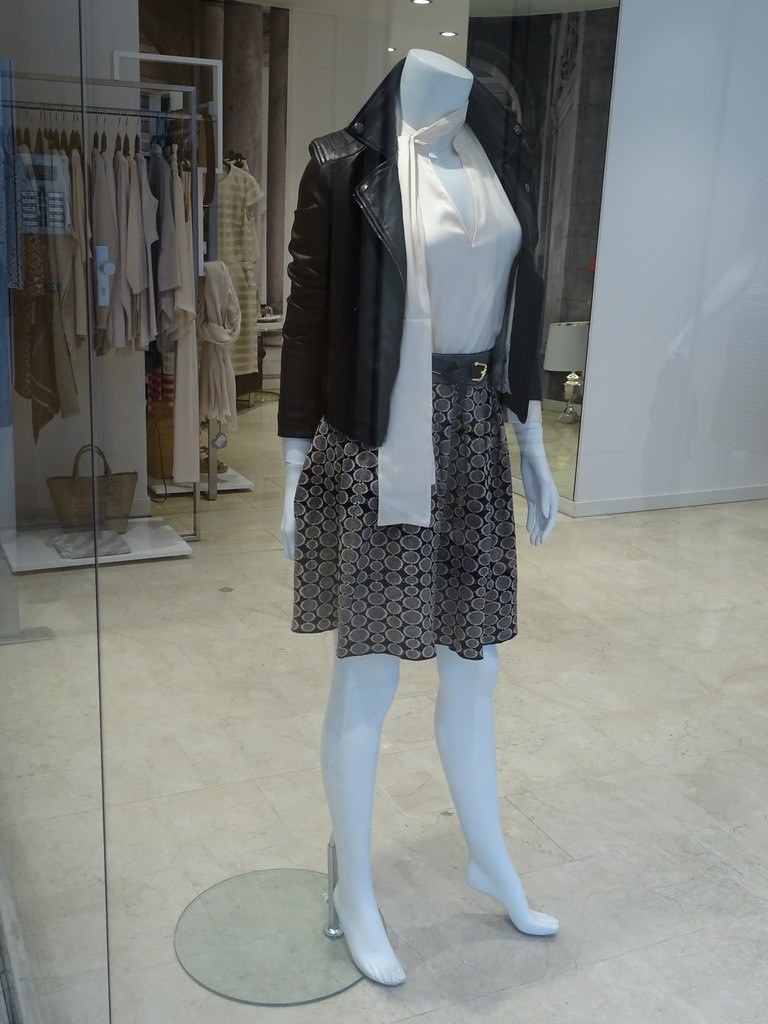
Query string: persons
[276,49,559,984]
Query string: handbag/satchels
[45,443,139,533]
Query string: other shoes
[200,457,229,473]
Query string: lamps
[545,323,588,423]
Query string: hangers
[17,100,246,171]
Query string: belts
[433,354,493,383]
